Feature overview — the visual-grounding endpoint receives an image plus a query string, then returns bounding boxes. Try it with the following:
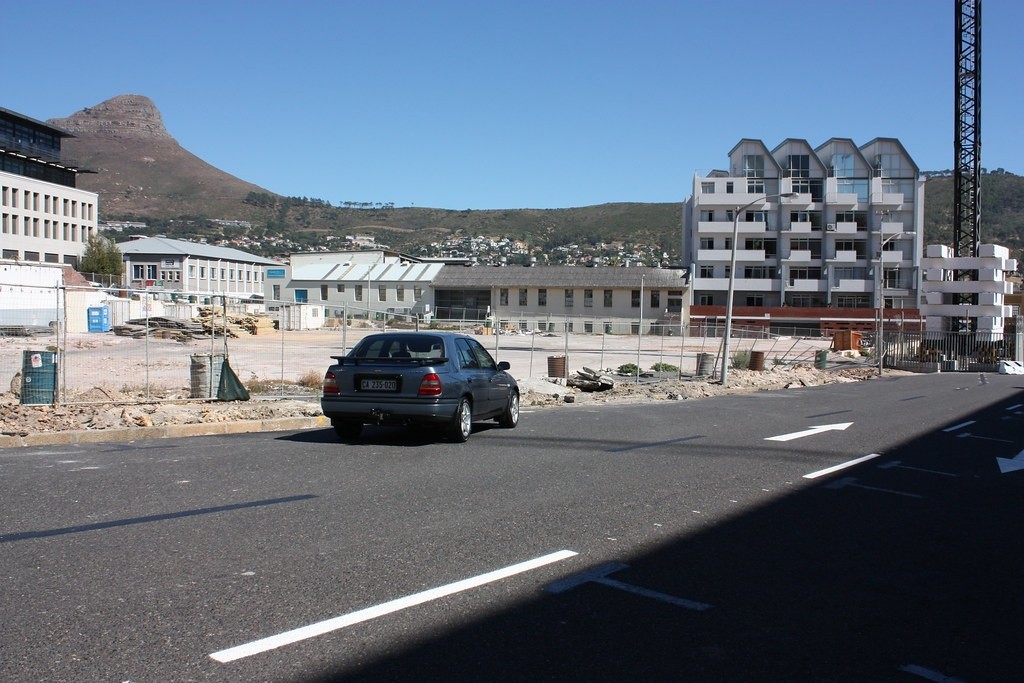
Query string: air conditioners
[826,223,836,231]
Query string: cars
[319,333,522,443]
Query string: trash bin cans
[696,353,715,376]
[749,352,764,371]
[548,355,569,378]
[814,350,827,370]
[190,355,225,398]
[20,350,56,404]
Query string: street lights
[719,190,800,385]
[878,231,918,376]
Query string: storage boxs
[87,305,111,333]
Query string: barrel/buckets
[548,355,569,378]
[696,353,714,376]
[750,351,764,370]
[190,352,225,398]
[19,349,56,406]
[814,351,826,369]
